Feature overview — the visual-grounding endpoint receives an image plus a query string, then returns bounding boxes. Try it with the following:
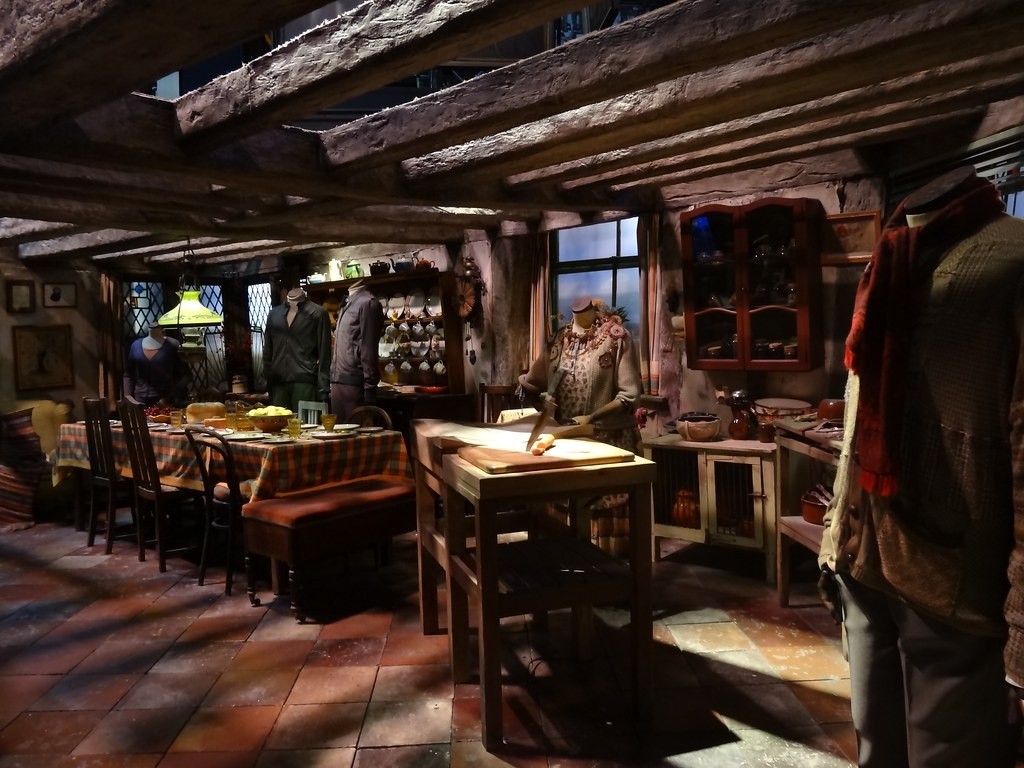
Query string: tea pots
[307,257,436,284]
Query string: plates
[76,419,168,427]
[166,429,195,433]
[300,423,316,428]
[378,329,445,358]
[354,427,383,433]
[317,423,360,430]
[148,426,172,430]
[374,287,442,320]
[223,433,272,440]
[200,430,234,436]
[414,385,448,393]
[309,430,357,438]
[262,439,293,443]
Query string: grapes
[144,405,180,417]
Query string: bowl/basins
[675,416,722,442]
[800,495,826,525]
[245,412,298,432]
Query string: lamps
[157,239,222,325]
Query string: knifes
[526,368,568,452]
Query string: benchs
[241,477,421,622]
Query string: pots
[796,399,846,432]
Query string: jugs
[225,400,255,431]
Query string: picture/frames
[43,282,78,308]
[436,19,556,67]
[14,324,74,390]
[6,278,36,313]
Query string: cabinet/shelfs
[303,269,461,388]
[680,200,827,371]
[642,433,776,584]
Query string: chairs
[182,428,255,595]
[347,406,391,435]
[297,401,330,428]
[80,395,134,553]
[121,395,203,573]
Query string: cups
[170,411,182,429]
[400,360,413,373]
[759,422,775,442]
[419,360,430,372]
[433,360,446,374]
[287,418,302,439]
[320,414,337,433]
[385,321,437,339]
[385,361,396,376]
[756,343,798,358]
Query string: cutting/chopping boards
[457,438,635,473]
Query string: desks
[412,417,657,755]
[57,422,415,591]
[776,416,844,610]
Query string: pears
[248,406,292,416]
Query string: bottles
[672,487,700,526]
[697,236,797,308]
